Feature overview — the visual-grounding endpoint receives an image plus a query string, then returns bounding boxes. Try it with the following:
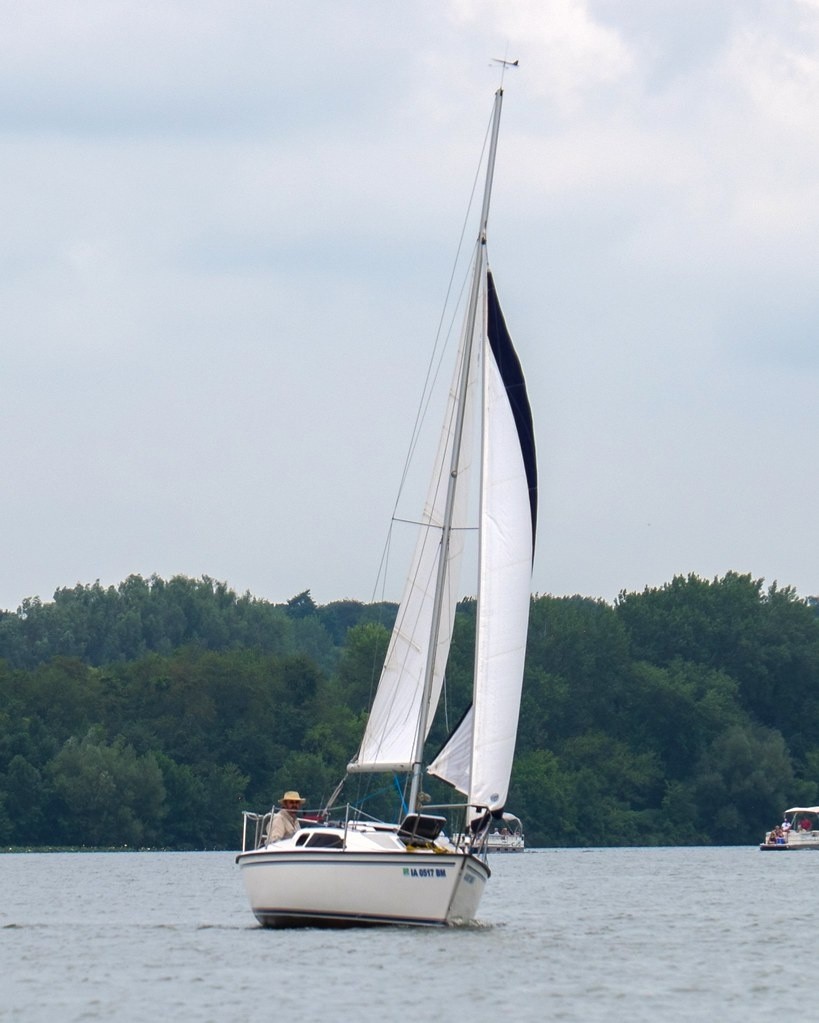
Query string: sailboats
[235,52,542,934]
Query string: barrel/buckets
[778,838,785,844]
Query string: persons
[768,815,810,844]
[263,791,305,851]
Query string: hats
[278,791,305,805]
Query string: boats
[450,812,525,854]
[758,805,819,850]
